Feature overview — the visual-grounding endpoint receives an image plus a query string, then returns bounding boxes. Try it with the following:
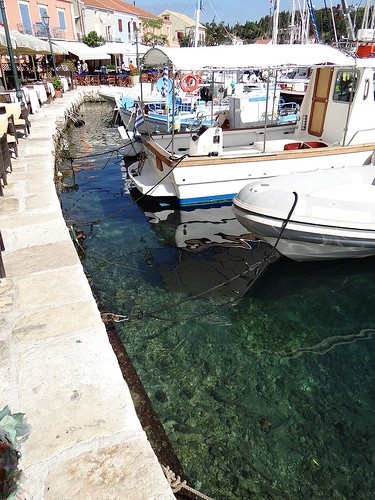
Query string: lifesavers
[179,74,199,93]
[193,73,202,86]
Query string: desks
[24,85,47,103]
[60,78,69,92]
[0,88,40,112]
[39,82,55,99]
[0,102,21,135]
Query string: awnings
[51,41,111,60]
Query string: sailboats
[97,0,375,169]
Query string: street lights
[42,11,59,79]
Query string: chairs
[0,75,135,283]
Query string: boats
[127,58,375,252]
[231,160,375,264]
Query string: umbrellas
[0,27,69,90]
[93,42,153,74]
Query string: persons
[129,61,134,72]
[82,59,88,72]
[77,61,81,71]
[121,62,125,69]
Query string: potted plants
[53,81,62,97]
